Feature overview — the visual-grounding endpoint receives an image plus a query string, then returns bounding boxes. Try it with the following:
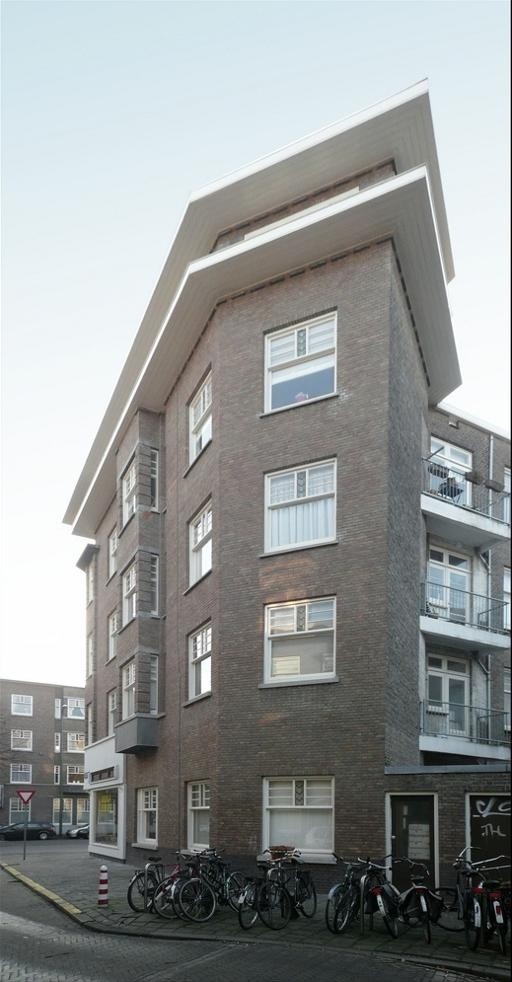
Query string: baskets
[269,846,295,864]
[362,860,385,875]
[343,855,367,870]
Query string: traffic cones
[97,865,109,908]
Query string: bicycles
[127,848,316,931]
[434,846,511,954]
[325,852,432,944]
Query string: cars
[66,824,90,839]
[0,821,56,841]
